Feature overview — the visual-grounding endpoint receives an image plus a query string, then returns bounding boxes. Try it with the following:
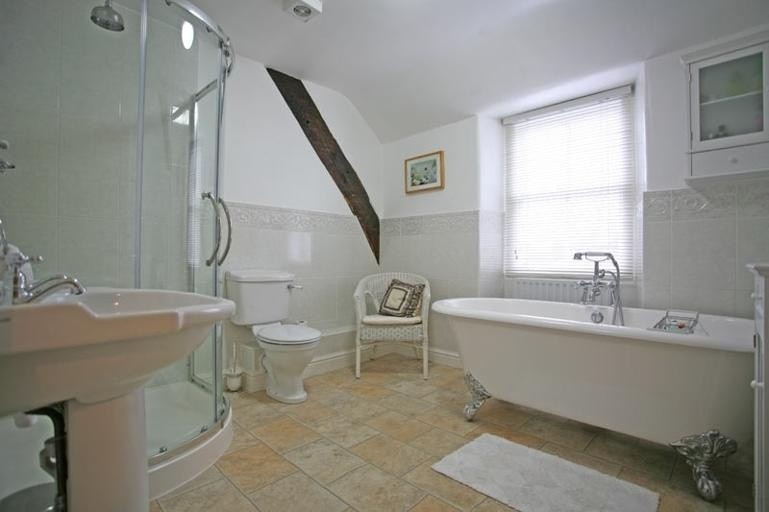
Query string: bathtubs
[431,295,755,454]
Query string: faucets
[0,251,86,305]
[579,259,615,303]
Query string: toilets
[225,268,324,405]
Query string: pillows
[378,279,426,319]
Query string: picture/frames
[404,151,446,193]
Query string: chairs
[352,271,431,380]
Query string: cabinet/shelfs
[679,30,768,186]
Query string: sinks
[1,284,236,412]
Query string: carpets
[430,432,661,511]
